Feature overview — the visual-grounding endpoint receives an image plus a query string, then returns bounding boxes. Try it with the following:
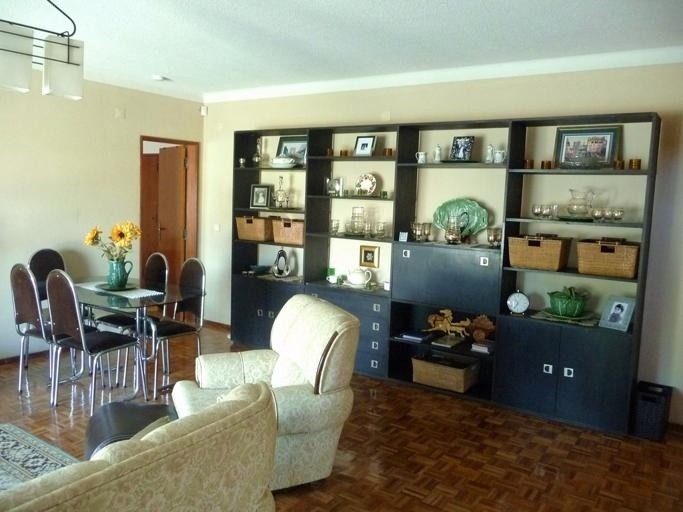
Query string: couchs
[0,381,278,512]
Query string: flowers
[84,222,142,261]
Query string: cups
[328,218,385,241]
[326,149,333,157]
[344,189,387,199]
[493,151,507,164]
[524,159,641,170]
[415,152,426,164]
[326,275,338,283]
[383,282,391,291]
[340,150,348,157]
[383,148,392,157]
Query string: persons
[609,304,624,322]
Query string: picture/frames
[276,136,307,168]
[249,184,270,210]
[351,135,376,159]
[553,125,624,171]
[599,295,636,332]
[360,245,380,269]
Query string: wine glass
[423,223,431,242]
[415,224,423,242]
[532,203,625,224]
[487,229,497,248]
[495,228,501,248]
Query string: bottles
[445,216,461,245]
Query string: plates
[355,174,376,195]
[270,163,296,168]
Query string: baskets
[272,218,304,245]
[235,216,280,243]
[508,234,571,272]
[576,239,640,279]
[411,349,482,394]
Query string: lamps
[0,0,86,98]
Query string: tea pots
[567,189,595,217]
[273,153,294,164]
[344,267,371,285]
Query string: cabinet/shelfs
[231,128,306,350]
[386,119,512,406]
[491,112,661,438]
[304,124,399,381]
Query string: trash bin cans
[633,380,675,444]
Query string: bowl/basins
[251,265,271,275]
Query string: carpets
[1,422,80,489]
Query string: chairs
[87,292,361,492]
[9,248,207,417]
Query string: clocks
[506,288,530,318]
